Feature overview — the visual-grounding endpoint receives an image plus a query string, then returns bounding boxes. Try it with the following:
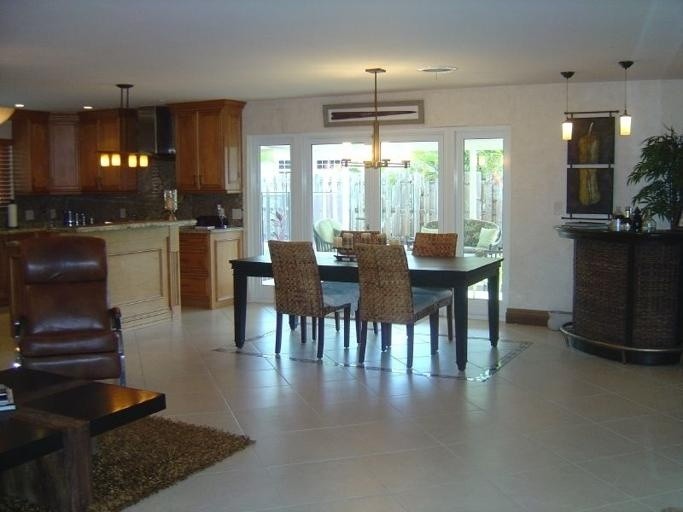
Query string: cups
[333,232,400,249]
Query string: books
[0,384,17,412]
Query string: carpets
[0,417,256,512]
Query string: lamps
[337,68,415,168]
[560,72,574,141]
[618,61,633,136]
[95,84,154,168]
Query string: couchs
[421,219,503,257]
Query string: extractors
[127,104,176,158]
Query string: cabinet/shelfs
[11,108,138,198]
[180,228,242,308]
[170,100,246,194]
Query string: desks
[0,367,166,512]
[229,251,504,371]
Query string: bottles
[64,209,94,228]
[216,203,227,229]
[610,205,657,233]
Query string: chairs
[406,233,458,348]
[353,242,453,368]
[268,240,351,359]
[335,231,387,341]
[313,218,343,252]
[11,233,127,386]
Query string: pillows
[421,227,438,234]
[477,228,497,249]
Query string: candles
[361,232,371,244]
[389,239,400,246]
[342,232,353,249]
[333,237,342,249]
